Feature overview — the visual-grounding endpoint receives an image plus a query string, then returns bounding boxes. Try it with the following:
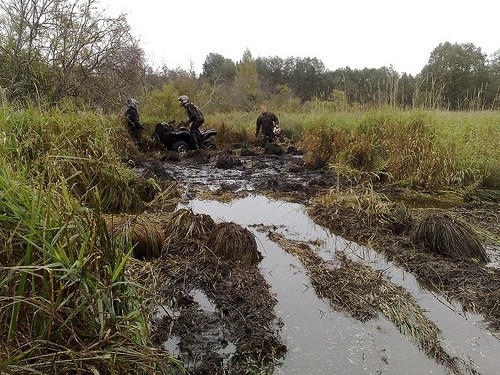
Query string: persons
[125,97,146,144]
[254,104,280,148]
[176,94,205,150]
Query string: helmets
[177,94,189,107]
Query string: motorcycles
[150,120,218,154]
